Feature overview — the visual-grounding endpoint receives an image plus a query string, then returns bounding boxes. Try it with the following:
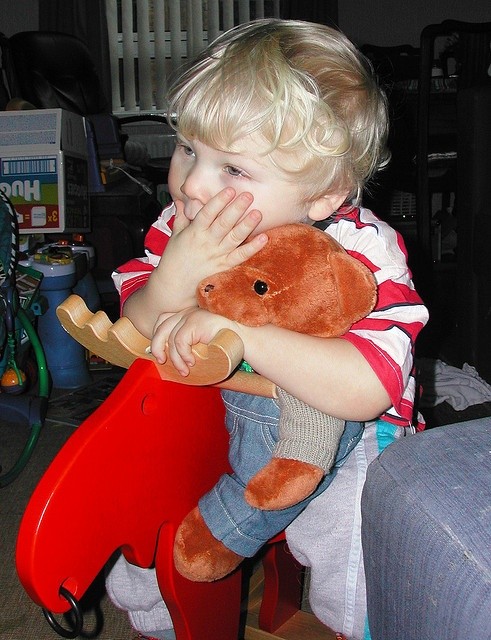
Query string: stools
[260,531,306,633]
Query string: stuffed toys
[173,223,379,584]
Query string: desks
[378,79,457,215]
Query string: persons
[103,17,430,639]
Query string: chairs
[361,42,421,86]
[382,19,490,283]
[1,31,176,186]
